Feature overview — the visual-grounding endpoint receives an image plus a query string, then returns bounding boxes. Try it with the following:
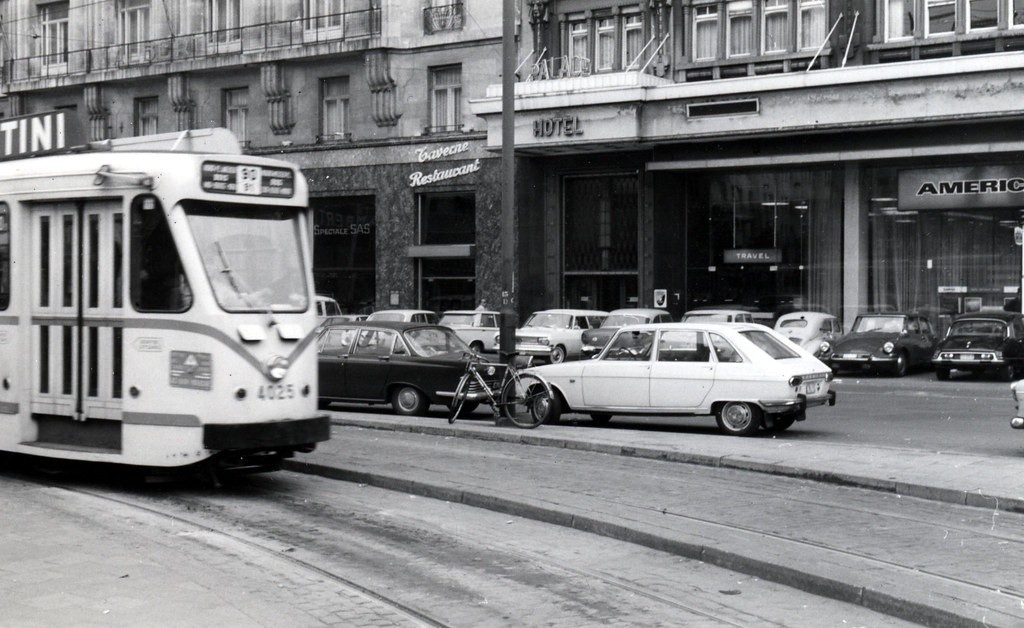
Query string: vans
[314,295,343,318]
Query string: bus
[0,109,328,488]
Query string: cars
[599,308,673,325]
[931,309,1024,380]
[515,308,608,364]
[437,310,500,355]
[314,320,501,416]
[516,322,836,436]
[818,312,938,377]
[366,309,439,324]
[317,314,369,326]
[774,311,842,364]
[680,308,754,325]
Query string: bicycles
[448,351,554,430]
